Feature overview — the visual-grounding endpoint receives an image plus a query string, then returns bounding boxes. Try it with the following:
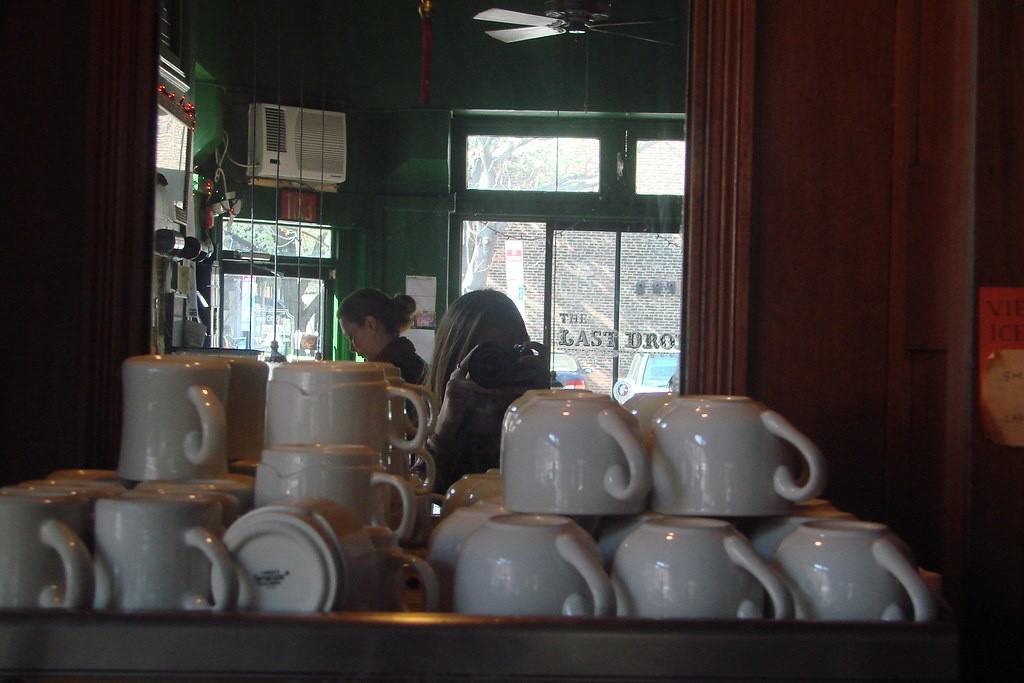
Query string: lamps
[264,13,288,381]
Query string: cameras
[467,342,534,389]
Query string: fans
[471,0,680,47]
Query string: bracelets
[428,431,456,448]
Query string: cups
[0,349,947,623]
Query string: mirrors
[80,0,758,548]
[151,89,195,226]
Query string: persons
[337,287,430,470]
[410,289,549,506]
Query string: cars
[621,347,681,405]
[533,349,592,390]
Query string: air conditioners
[247,103,346,184]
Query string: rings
[457,363,469,373]
